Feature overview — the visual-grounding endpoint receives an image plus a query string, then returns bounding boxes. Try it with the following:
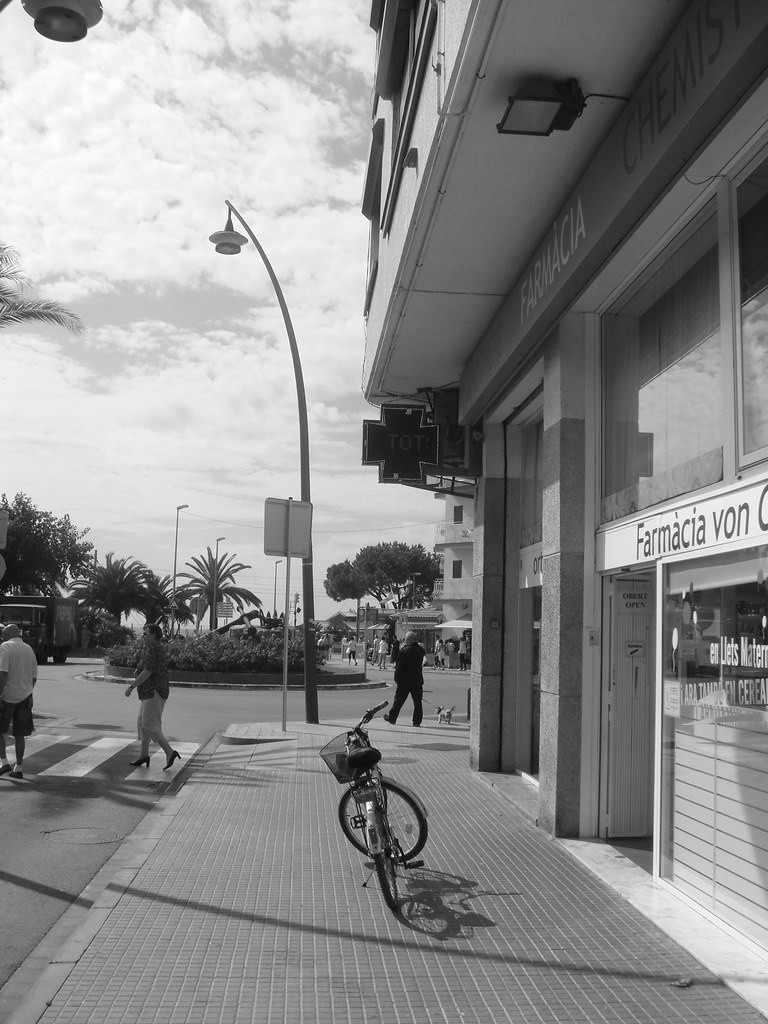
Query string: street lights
[413,572,421,610]
[209,199,320,723]
[274,560,282,619]
[170,505,189,637]
[213,537,226,629]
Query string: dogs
[434,705,456,724]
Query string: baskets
[319,729,369,784]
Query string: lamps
[497,94,585,137]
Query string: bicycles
[319,701,429,910]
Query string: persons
[317,632,334,660]
[370,634,405,669]
[431,634,467,670]
[418,638,424,649]
[0,624,38,780]
[383,631,426,726]
[124,623,182,771]
[341,635,363,666]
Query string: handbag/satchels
[346,647,350,654]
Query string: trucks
[0,596,79,665]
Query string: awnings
[434,614,472,631]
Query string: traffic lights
[297,608,301,613]
[294,593,300,604]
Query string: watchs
[129,684,134,689]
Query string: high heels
[162,750,181,770]
[130,756,150,767]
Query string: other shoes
[9,771,23,779]
[383,714,395,724]
[463,668,466,671]
[0,764,12,776]
[458,668,462,671]
[412,720,421,727]
[432,666,445,671]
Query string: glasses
[143,632,150,636]
